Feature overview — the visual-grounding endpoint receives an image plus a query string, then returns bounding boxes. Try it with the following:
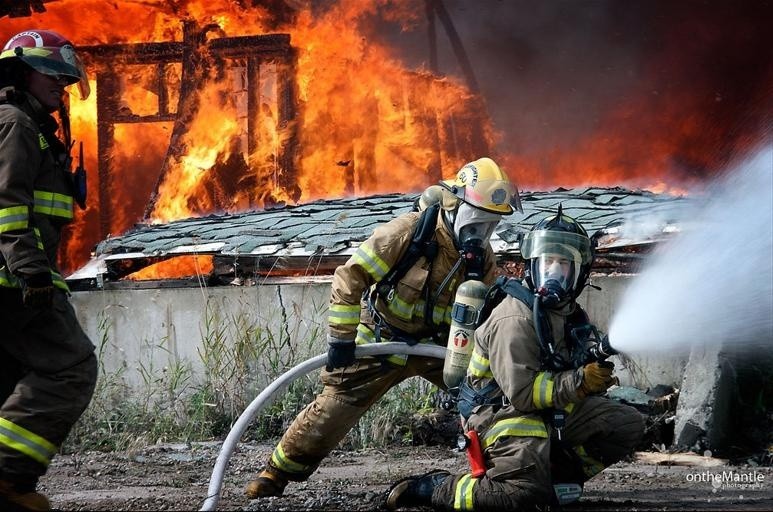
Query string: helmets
[520,212,595,265]
[439,158,522,215]
[1,29,91,76]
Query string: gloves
[575,361,619,399]
[22,271,54,312]
[325,342,356,372]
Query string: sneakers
[248,469,287,498]
[385,469,450,510]
[1,482,51,512]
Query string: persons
[243,157,522,500]
[0,28,100,511]
[383,212,658,511]
